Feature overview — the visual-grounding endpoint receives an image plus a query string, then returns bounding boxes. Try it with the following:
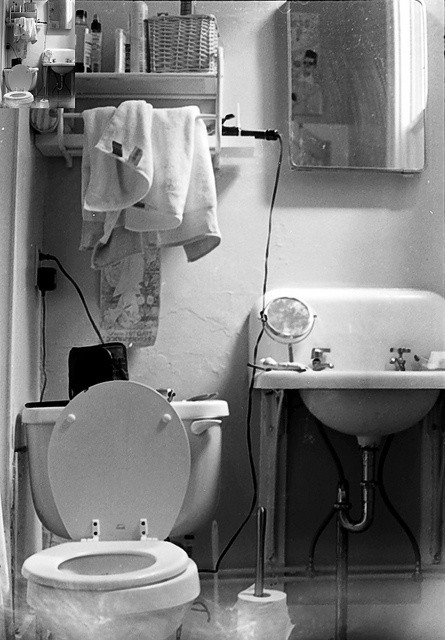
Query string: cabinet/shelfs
[32,45,224,172]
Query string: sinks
[249,368,445,449]
[43,62,75,75]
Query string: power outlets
[33,243,47,291]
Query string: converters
[37,264,58,294]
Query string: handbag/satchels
[69,341,128,399]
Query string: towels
[126,106,222,261]
[78,100,155,271]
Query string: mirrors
[284,0,428,175]
[260,294,315,365]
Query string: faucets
[311,348,333,371]
[52,57,56,63]
[389,348,411,370]
[65,58,71,63]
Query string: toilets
[20,380,230,640]
[3,64,39,108]
